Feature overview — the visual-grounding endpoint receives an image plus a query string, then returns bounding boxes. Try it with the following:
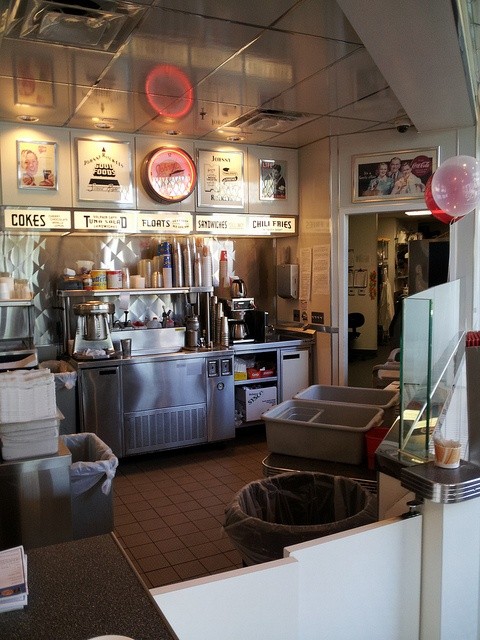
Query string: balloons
[426,165,473,224]
[432,155,480,217]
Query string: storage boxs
[292,384,400,429]
[260,398,385,465]
[236,386,278,423]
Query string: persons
[369,163,393,195]
[264,165,284,198]
[387,157,403,180]
[22,151,53,186]
[392,163,426,195]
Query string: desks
[260,331,470,495]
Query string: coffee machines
[227,297,256,343]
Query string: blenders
[72,300,118,360]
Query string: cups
[434,440,462,469]
[208,296,229,347]
[123,234,232,289]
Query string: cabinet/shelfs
[234,345,314,430]
[396,243,408,298]
[56,286,237,459]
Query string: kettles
[231,278,247,297]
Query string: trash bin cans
[59,433,120,540]
[222,471,374,567]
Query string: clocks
[140,62,193,124]
[141,145,198,205]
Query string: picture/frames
[193,65,243,135]
[198,148,245,209]
[16,139,59,192]
[259,158,289,200]
[12,53,57,110]
[74,136,133,205]
[70,44,131,123]
[350,146,441,203]
